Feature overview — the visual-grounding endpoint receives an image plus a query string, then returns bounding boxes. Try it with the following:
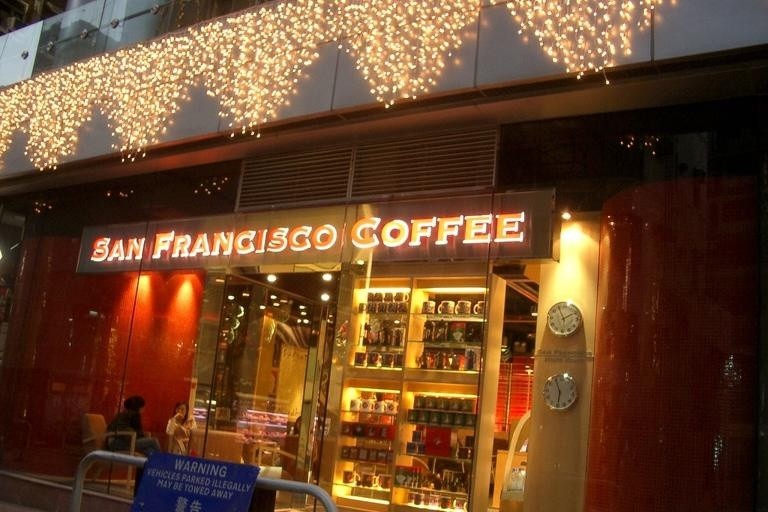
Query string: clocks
[543,300,583,412]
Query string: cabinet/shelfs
[313,273,506,511]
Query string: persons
[105,394,161,458]
[283,415,301,436]
[166,400,196,456]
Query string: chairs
[79,413,147,490]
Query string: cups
[408,492,466,511]
[350,398,398,415]
[354,292,485,372]
[343,470,392,490]
